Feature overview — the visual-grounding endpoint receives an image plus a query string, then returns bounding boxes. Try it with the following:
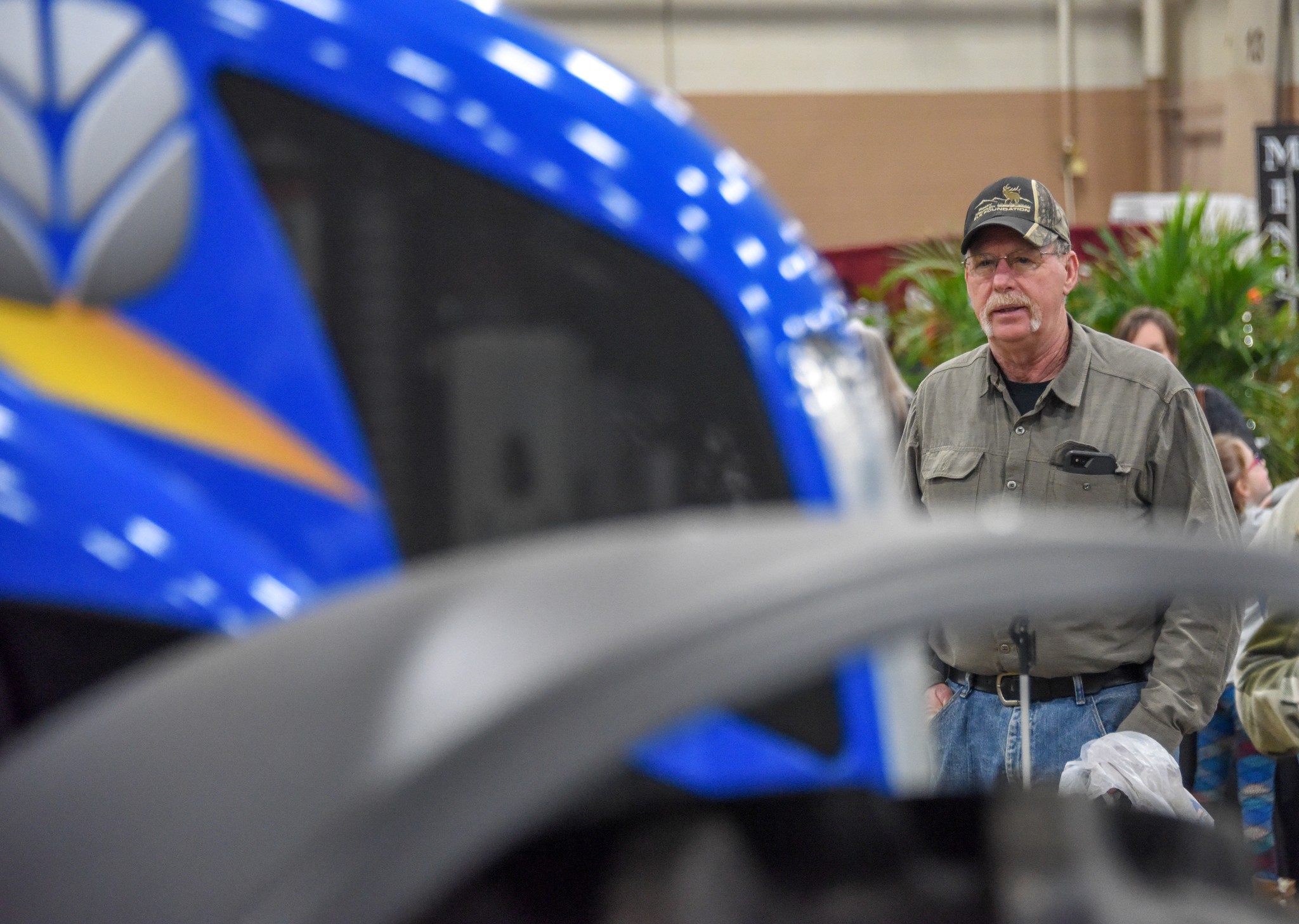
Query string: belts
[944,664,1155,706]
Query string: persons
[1108,306,1256,444]
[1188,434,1280,908]
[898,178,1247,827]
[842,316,916,440]
[1233,605,1299,754]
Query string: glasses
[962,248,1066,278]
[1243,451,1264,476]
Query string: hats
[962,177,1072,251]
